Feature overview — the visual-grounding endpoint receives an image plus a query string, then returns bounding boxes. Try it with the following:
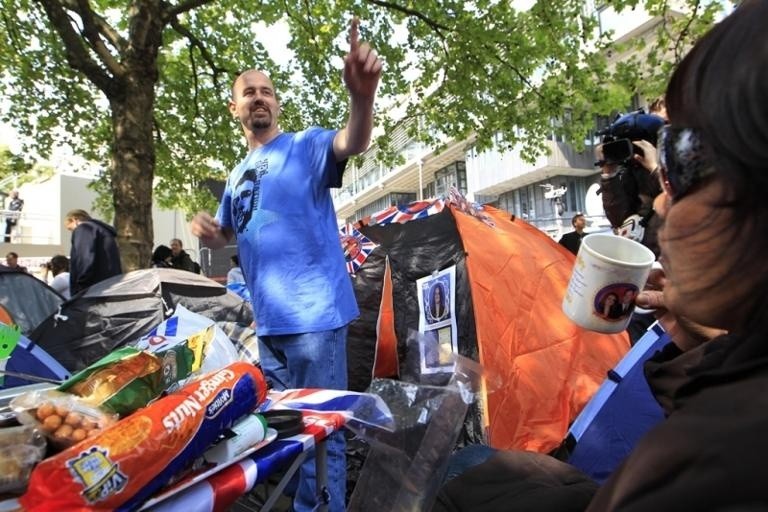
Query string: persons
[231,168,257,233]
[6,252,28,273]
[4,187,25,243]
[190,17,382,511]
[226,255,245,284]
[170,238,201,274]
[425,1,767,512]
[594,111,668,262]
[64,208,123,298]
[559,212,591,256]
[431,286,444,318]
[41,254,72,302]
[598,288,636,319]
[152,245,174,268]
[646,92,671,125]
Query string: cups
[560,233,665,334]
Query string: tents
[554,320,675,484]
[1,264,265,390]
[1,264,71,338]
[0,323,72,390]
[235,187,655,460]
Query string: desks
[146,386,376,511]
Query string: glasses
[655,117,729,204]
